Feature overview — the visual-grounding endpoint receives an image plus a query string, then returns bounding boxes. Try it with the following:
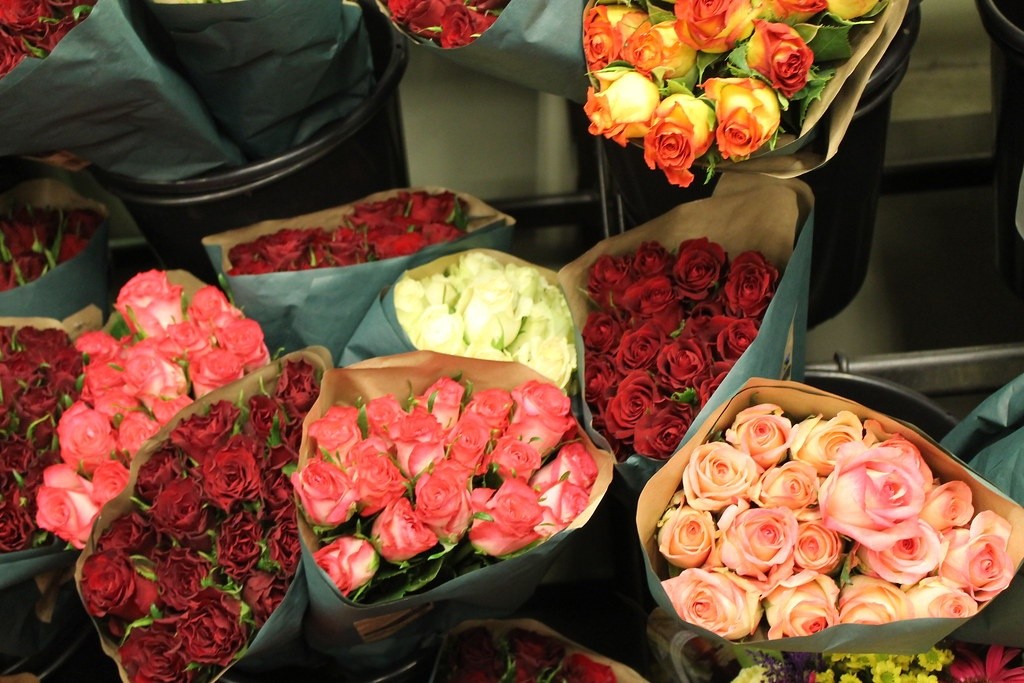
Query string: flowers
[728,643,1024,683]
[386,0,505,49]
[225,189,473,275]
[582,0,890,187]
[290,368,599,603]
[0,326,90,554]
[78,356,324,683]
[0,201,103,292]
[394,250,589,395]
[445,626,618,683]
[0,0,98,79]
[576,235,781,463]
[652,400,1012,640]
[35,269,271,552]
